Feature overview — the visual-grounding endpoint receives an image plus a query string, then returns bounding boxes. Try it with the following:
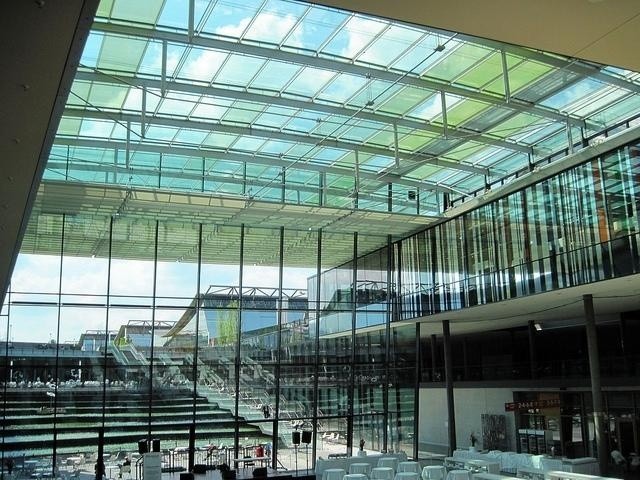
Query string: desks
[317,447,614,479]
[12,442,291,480]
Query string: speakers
[253,467,267,477]
[292,432,300,444]
[180,473,194,480]
[194,465,206,473]
[222,470,236,480]
[153,439,160,452]
[302,431,312,443]
[139,439,147,454]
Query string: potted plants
[468,433,478,452]
[358,439,366,457]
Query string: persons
[609,447,640,480]
[118,456,132,479]
[261,402,271,419]
[254,443,265,464]
[202,441,217,462]
